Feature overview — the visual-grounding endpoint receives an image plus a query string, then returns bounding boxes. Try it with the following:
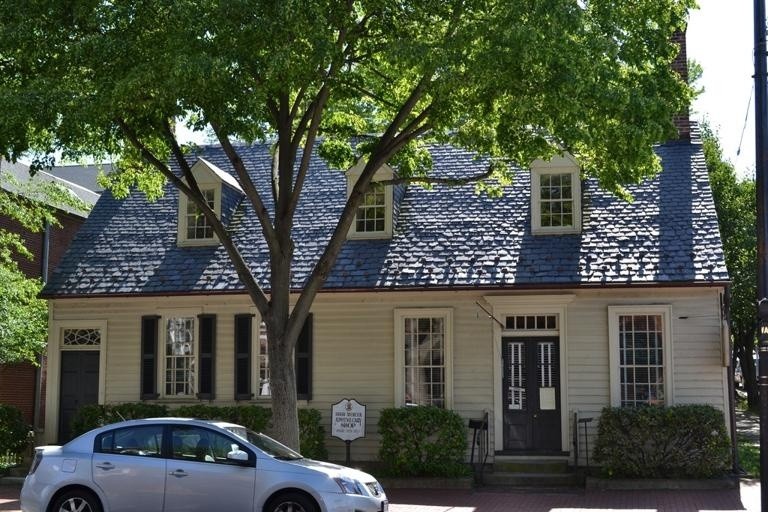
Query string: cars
[18,417,391,512]
